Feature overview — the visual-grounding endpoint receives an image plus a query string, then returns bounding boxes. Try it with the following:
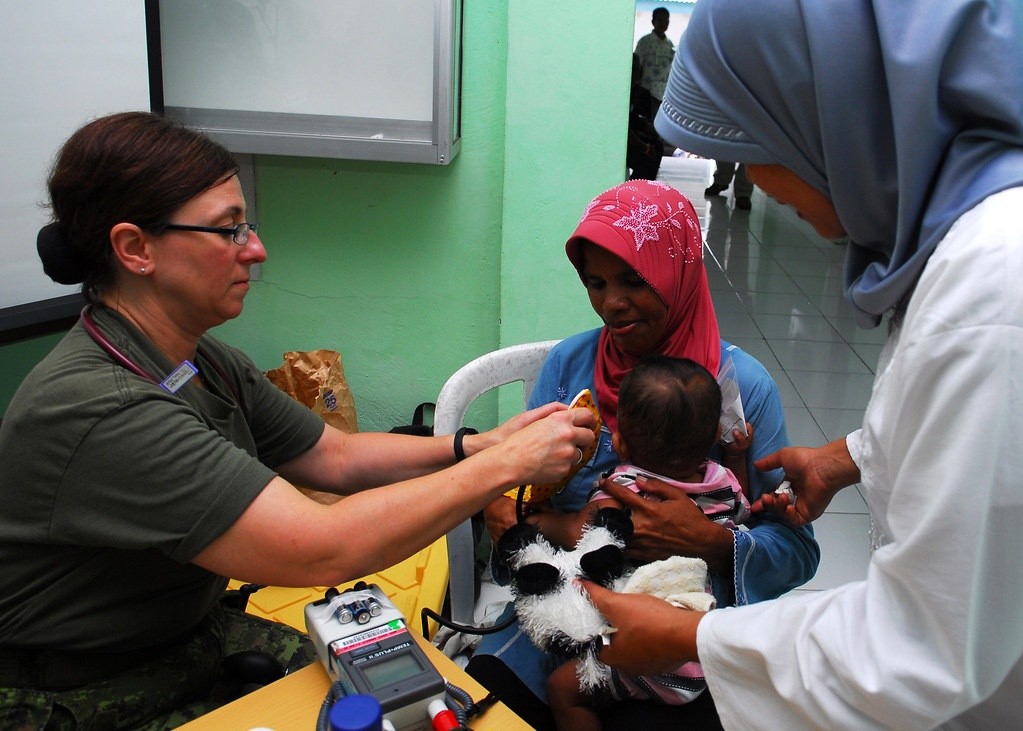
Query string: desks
[167,622,540,731]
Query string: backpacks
[388,402,436,437]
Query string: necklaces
[81,305,162,387]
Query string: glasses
[132,223,261,245]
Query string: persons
[522,356,752,731]
[704,160,754,210]
[0,111,597,731]
[465,179,821,731]
[573,0,1023,731]
[626,8,676,181]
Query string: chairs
[430,339,562,628]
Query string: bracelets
[454,427,479,462]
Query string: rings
[573,448,583,465]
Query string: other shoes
[705,181,729,197]
[736,196,752,209]
